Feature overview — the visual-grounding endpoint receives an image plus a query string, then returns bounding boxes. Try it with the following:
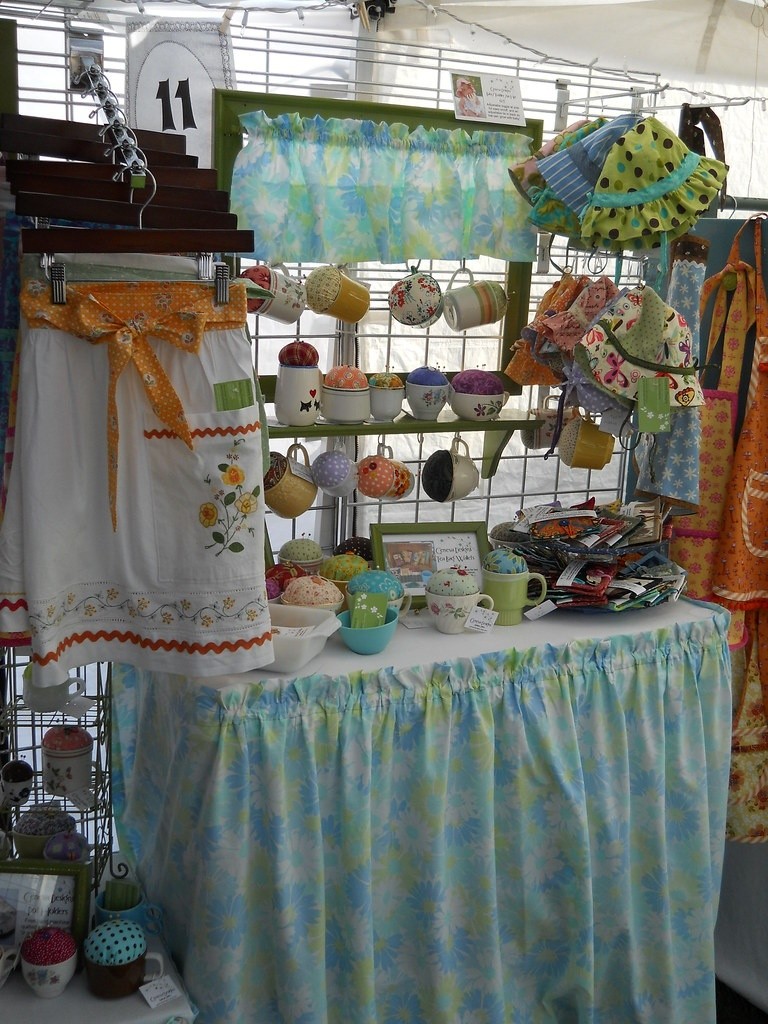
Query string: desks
[0,852,198,1024]
[106,593,734,1024]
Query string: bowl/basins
[448,391,510,421]
[337,607,398,656]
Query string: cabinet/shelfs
[0,646,131,904]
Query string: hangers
[0,64,256,306]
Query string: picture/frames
[0,860,92,973]
[369,521,491,609]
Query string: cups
[94,892,163,937]
[265,537,547,643]
[369,385,405,421]
[387,264,444,329]
[321,384,371,425]
[520,394,580,450]
[263,444,318,519]
[19,928,79,998]
[240,262,307,325]
[312,441,360,497]
[422,437,479,503]
[23,660,85,713]
[274,365,323,426]
[357,443,416,501]
[442,268,508,332]
[558,405,616,471]
[405,380,450,419]
[84,920,164,998]
[305,264,371,324]
[41,725,103,796]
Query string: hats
[506,111,730,432]
